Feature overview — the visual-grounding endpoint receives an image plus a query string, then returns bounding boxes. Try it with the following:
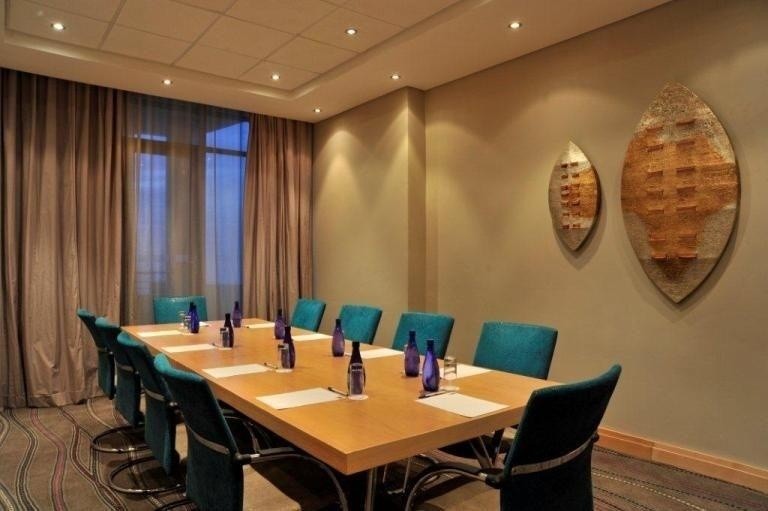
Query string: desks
[120,317,566,511]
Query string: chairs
[402,361,622,511]
[107,331,261,495]
[75,308,145,401]
[89,316,185,455]
[402,319,558,500]
[152,295,208,324]
[153,351,350,511]
[337,304,383,345]
[289,298,326,332]
[381,311,455,497]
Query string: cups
[276,344,290,372]
[349,364,366,401]
[219,328,230,350]
[443,356,459,392]
[176,311,191,333]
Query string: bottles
[224,313,234,348]
[232,301,241,327]
[403,331,420,378]
[275,309,285,339]
[346,342,366,395]
[281,327,295,368]
[332,319,345,357]
[421,340,440,391]
[187,302,199,334]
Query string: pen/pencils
[327,385,349,397]
[264,362,279,370]
[418,391,446,399]
[242,326,250,328]
[212,343,223,349]
